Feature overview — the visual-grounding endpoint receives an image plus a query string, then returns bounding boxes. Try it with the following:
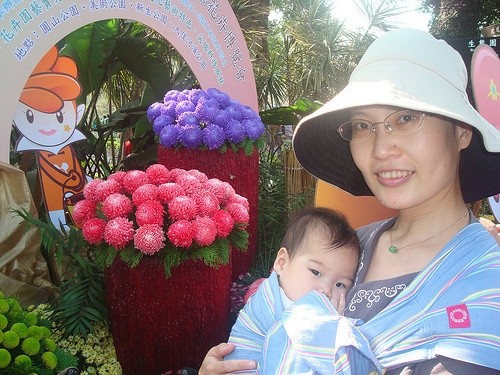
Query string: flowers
[70,164,249,279]
[147,88,266,156]
[28,303,122,375]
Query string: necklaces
[389,211,469,253]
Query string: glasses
[336,110,429,142]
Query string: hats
[291,29,500,204]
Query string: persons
[198,28,500,375]
[224,207,361,375]
